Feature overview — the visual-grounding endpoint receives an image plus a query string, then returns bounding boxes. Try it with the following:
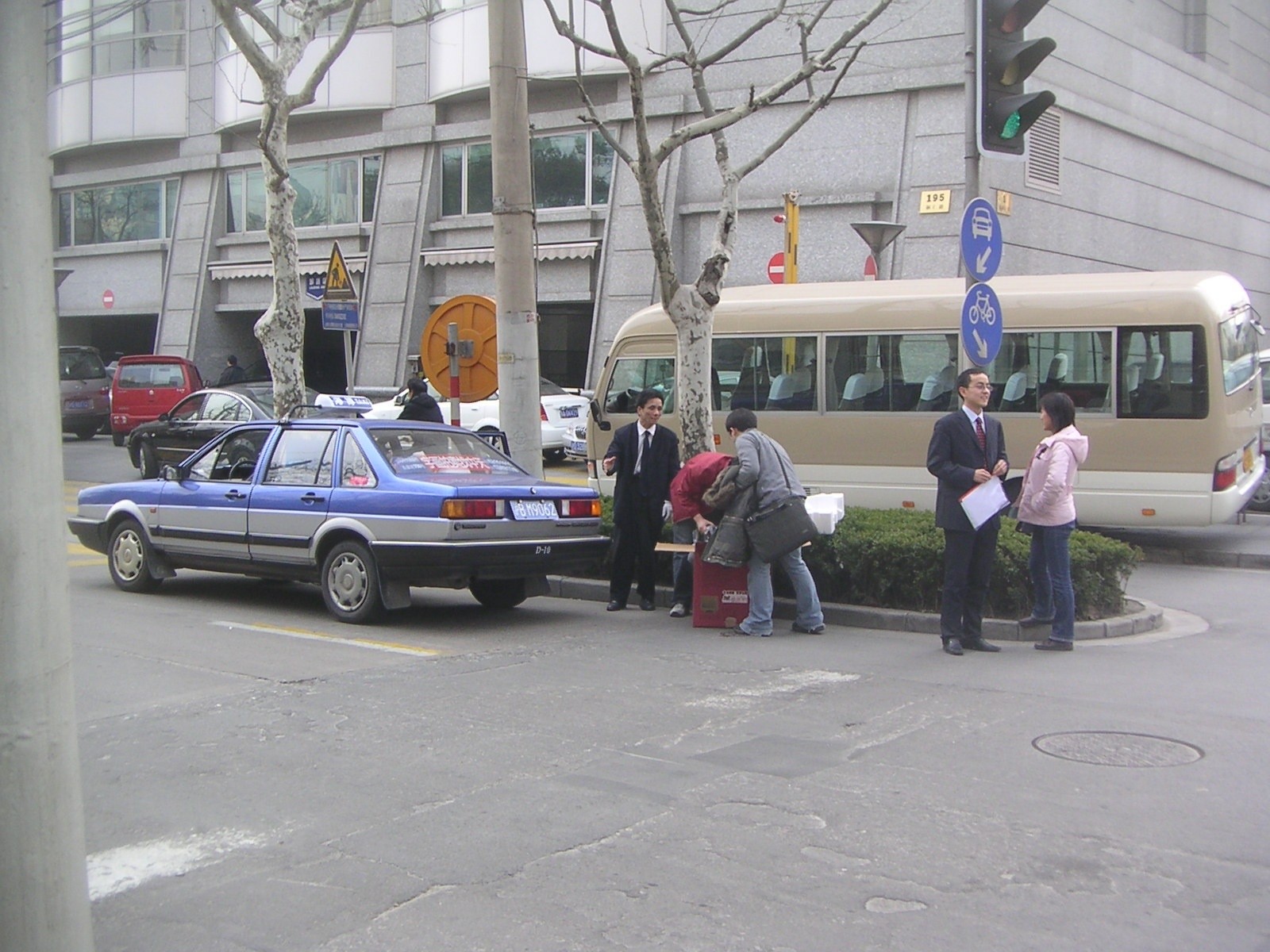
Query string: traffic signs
[321,301,361,332]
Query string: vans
[112,355,211,445]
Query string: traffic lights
[976,0,1059,162]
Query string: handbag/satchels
[740,492,821,565]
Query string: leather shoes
[638,597,656,610]
[961,632,1002,653]
[605,599,625,613]
[942,635,965,658]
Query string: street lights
[850,221,907,279]
[53,267,75,344]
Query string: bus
[589,270,1265,552]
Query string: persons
[927,368,1009,655]
[217,355,248,388]
[376,378,449,457]
[603,388,680,610]
[1010,392,1088,651]
[725,410,826,636]
[669,452,739,617]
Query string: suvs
[57,345,110,438]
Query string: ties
[641,429,651,499]
[975,415,988,456]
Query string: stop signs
[102,289,115,311]
[769,253,786,283]
[863,254,881,280]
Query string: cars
[126,381,358,479]
[358,364,588,465]
[563,417,590,460]
[68,392,605,624]
[1249,346,1270,512]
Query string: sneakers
[1034,638,1076,652]
[670,601,689,617]
[790,619,825,635]
[733,620,771,638]
[1018,612,1054,627]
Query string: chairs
[1223,352,1256,393]
[1045,353,1068,393]
[1020,365,1038,412]
[916,374,944,412]
[766,375,794,410]
[997,372,1026,412]
[941,365,956,412]
[1103,353,1165,415]
[790,367,812,409]
[866,366,885,411]
[836,374,867,410]
[730,347,763,409]
